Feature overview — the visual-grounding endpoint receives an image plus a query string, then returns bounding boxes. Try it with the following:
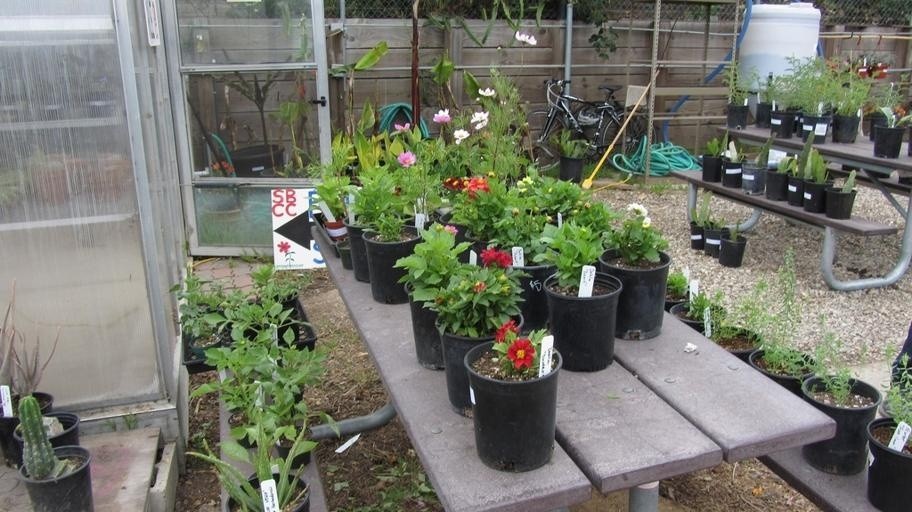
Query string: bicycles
[512,78,657,175]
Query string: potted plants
[665,271,687,315]
[396,224,471,370]
[702,136,855,219]
[802,310,883,474]
[490,211,557,330]
[701,292,762,359]
[722,53,912,159]
[19,395,93,512]
[544,218,622,376]
[747,249,819,393]
[169,249,328,511]
[867,341,910,512]
[674,287,725,329]
[424,267,524,417]
[690,190,747,267]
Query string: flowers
[491,317,552,371]
[306,35,670,305]
[212,162,233,177]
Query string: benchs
[826,166,910,197]
[672,169,895,289]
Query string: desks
[313,226,836,512]
[718,123,912,289]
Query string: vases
[204,203,250,230]
[0,391,53,464]
[599,246,670,341]
[459,340,563,474]
[15,413,80,466]
